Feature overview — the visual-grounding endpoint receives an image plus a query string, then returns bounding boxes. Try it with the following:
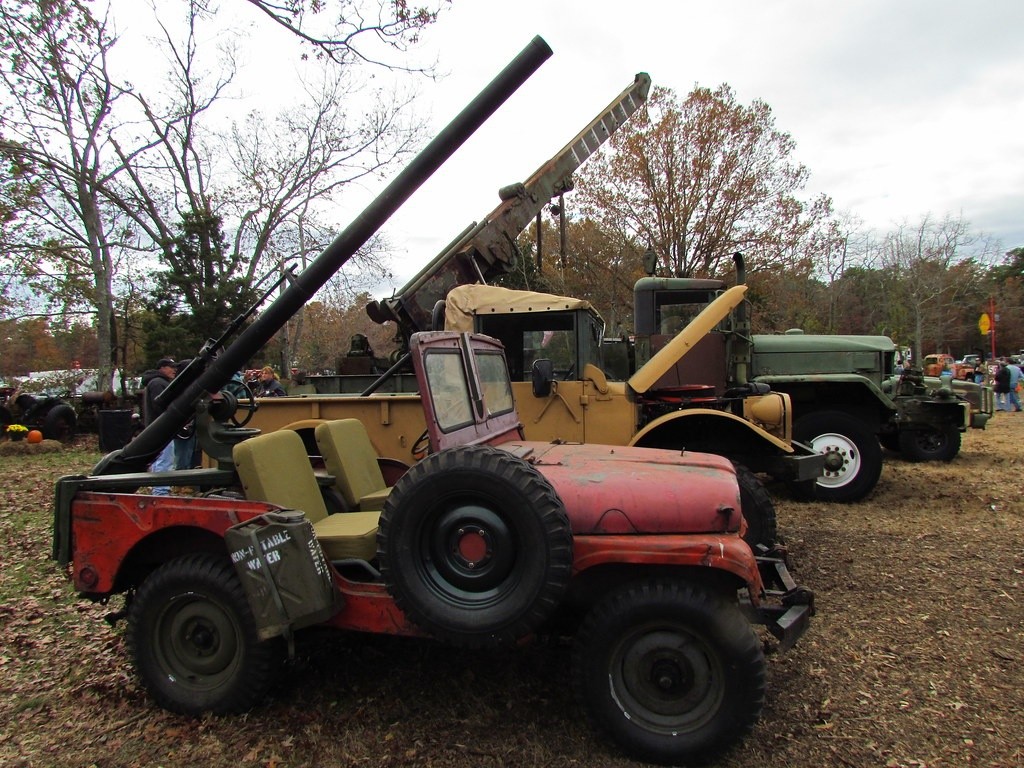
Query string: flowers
[7,423,28,434]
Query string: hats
[156,358,181,370]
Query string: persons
[893,361,904,374]
[938,351,1024,412]
[223,368,249,400]
[243,369,265,399]
[172,360,200,496]
[256,365,287,397]
[291,367,319,385]
[141,358,178,496]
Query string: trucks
[50,33,994,768]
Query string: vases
[11,432,25,441]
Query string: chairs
[314,417,393,511]
[232,428,383,560]
